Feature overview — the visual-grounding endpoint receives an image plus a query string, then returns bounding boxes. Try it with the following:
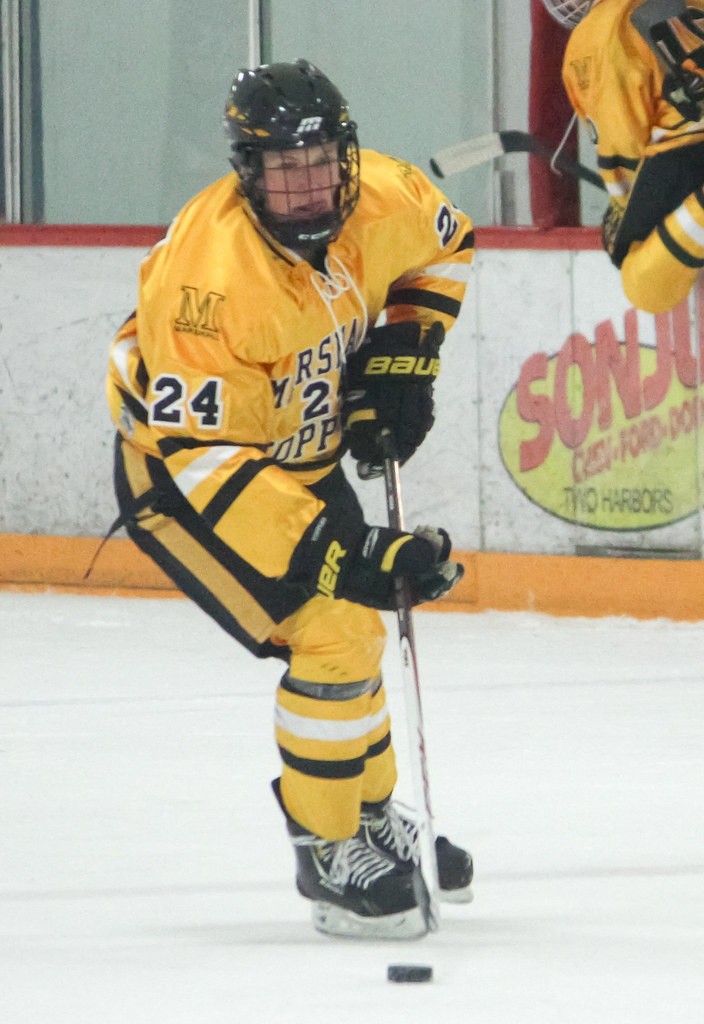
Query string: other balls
[385,963,435,986]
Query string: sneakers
[271,776,430,940]
[355,791,475,902]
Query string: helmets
[224,58,360,247]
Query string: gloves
[305,511,464,611]
[338,322,445,480]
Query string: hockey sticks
[428,128,607,196]
[373,416,443,935]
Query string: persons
[527,1,704,316]
[103,56,475,936]
